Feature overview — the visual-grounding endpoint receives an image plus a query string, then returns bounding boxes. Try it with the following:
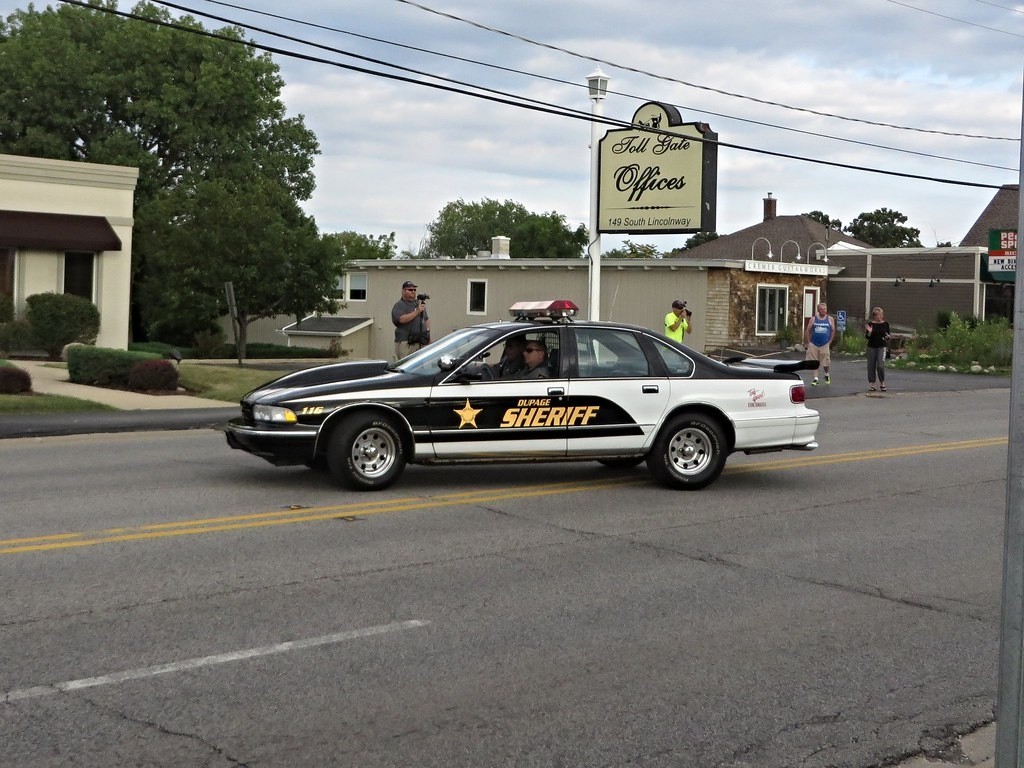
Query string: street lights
[585,65,613,365]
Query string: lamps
[780,239,802,262]
[751,237,773,260]
[928,276,940,287]
[894,274,905,287]
[806,242,828,264]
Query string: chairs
[548,348,559,378]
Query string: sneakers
[811,380,819,386]
[825,375,830,384]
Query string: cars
[224,299,822,489]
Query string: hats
[403,281,418,288]
[672,300,683,307]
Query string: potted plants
[772,325,798,351]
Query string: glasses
[675,306,683,309]
[405,289,416,291]
[524,348,540,353]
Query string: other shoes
[880,385,886,392]
[869,386,876,391]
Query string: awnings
[0,208,122,252]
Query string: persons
[391,280,430,360]
[864,307,892,392]
[662,299,693,362]
[463,334,551,381]
[805,302,835,386]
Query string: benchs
[610,360,687,377]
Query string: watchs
[426,328,430,331]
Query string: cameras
[417,293,430,302]
[684,309,692,317]
